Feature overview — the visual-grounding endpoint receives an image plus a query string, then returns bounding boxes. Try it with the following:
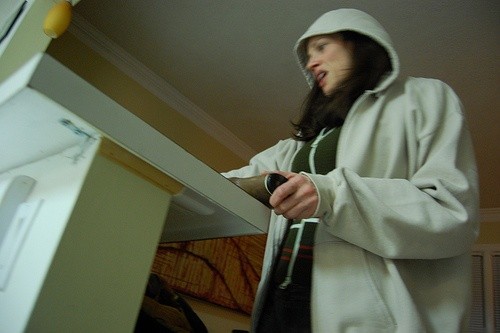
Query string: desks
[0,52,272,333]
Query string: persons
[217,6,479,331]
